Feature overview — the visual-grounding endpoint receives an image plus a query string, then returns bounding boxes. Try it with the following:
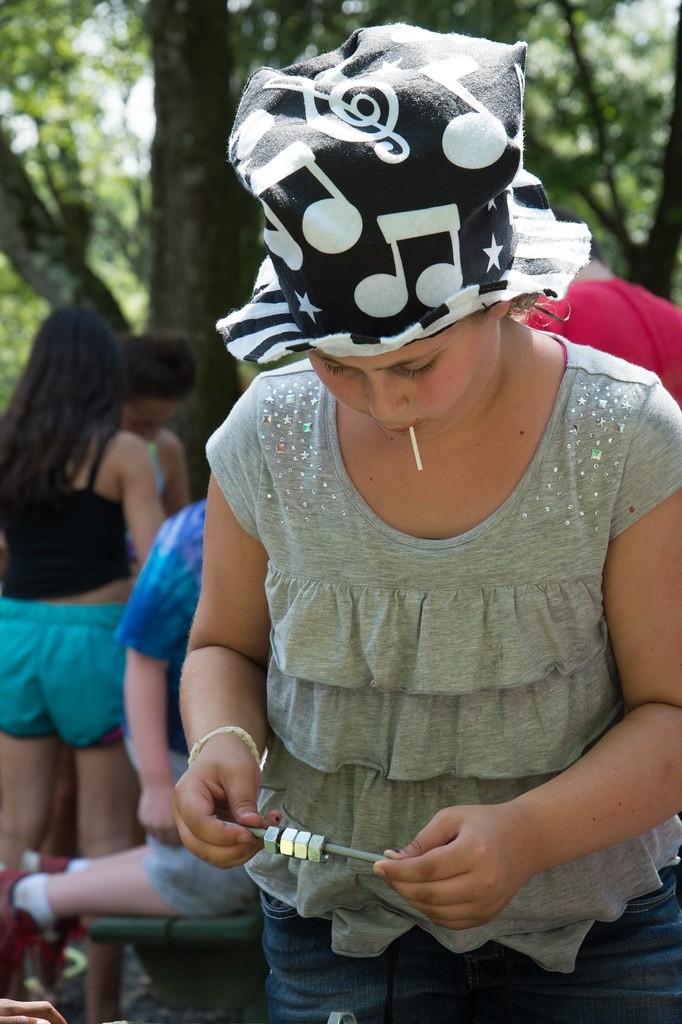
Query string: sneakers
[0,863,47,999]
[21,847,86,993]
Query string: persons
[0,996,69,1024]
[509,206,682,407]
[16,332,198,1004]
[0,495,262,1006]
[0,300,171,1024]
[171,22,681,1023]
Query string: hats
[216,19,595,367]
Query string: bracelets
[187,723,261,773]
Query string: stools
[78,909,263,1024]
